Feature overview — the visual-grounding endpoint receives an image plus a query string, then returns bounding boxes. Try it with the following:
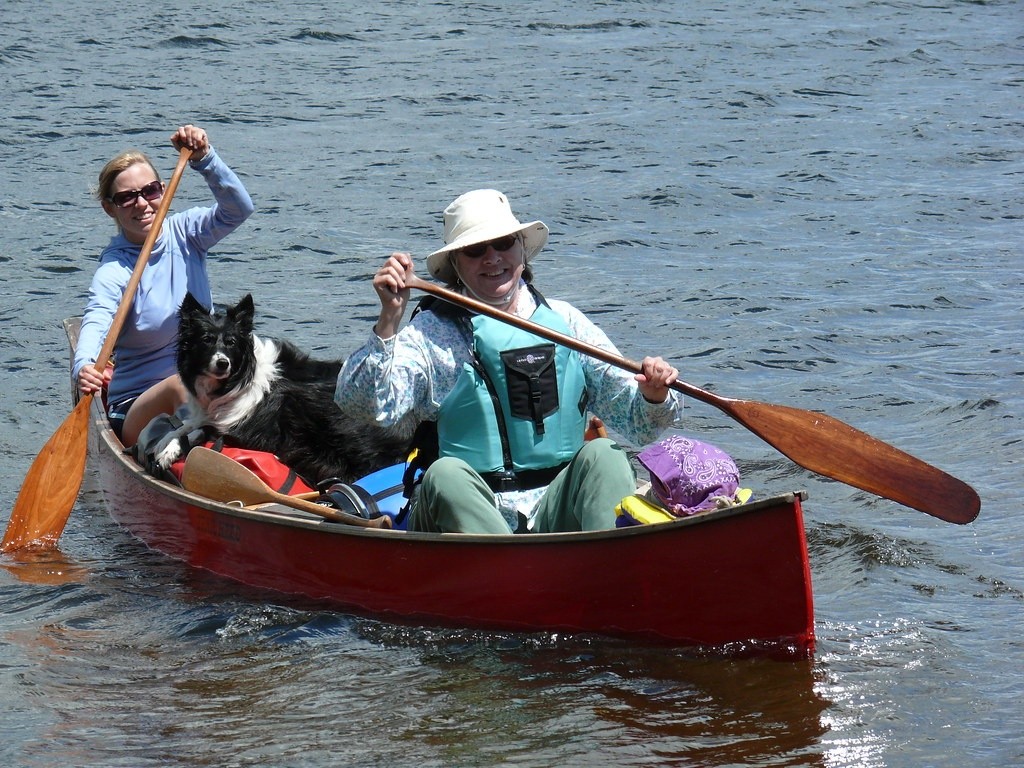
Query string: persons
[334,188,682,533]
[69,125,253,446]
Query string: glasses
[458,235,518,258]
[104,180,162,209]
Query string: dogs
[143,291,421,484]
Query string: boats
[56,312,823,657]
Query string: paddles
[2,144,190,549]
[181,446,393,529]
[402,276,980,528]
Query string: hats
[426,189,549,285]
